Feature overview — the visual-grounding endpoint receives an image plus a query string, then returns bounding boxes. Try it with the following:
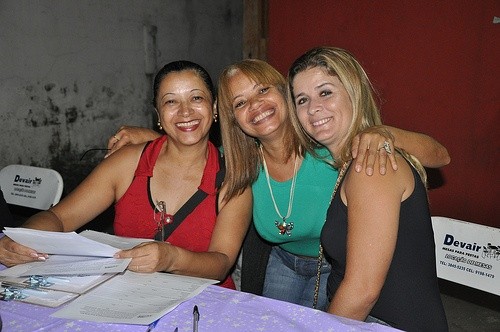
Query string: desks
[0,263,407,332]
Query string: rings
[379,141,391,154]
[114,135,119,141]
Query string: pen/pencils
[147,320,158,332]
[193,305,200,332]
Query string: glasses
[153,200,166,245]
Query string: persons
[288,46,449,332]
[0,60,254,291]
[105,58,451,310]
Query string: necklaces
[260,145,301,237]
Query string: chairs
[0,164,65,233]
[431,216,500,296]
